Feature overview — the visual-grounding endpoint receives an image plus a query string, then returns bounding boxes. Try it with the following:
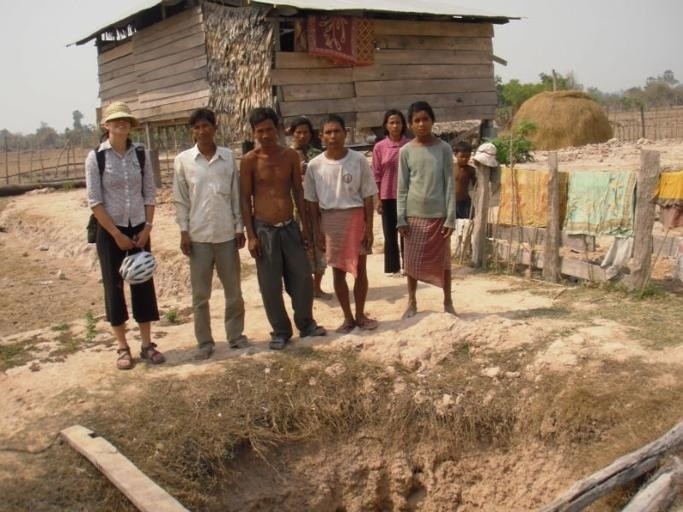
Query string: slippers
[354,318,377,329]
[335,323,354,334]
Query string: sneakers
[303,320,325,335]
[194,342,212,360]
[229,336,249,347]
[269,336,288,349]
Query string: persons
[302,115,380,333]
[287,117,333,300]
[294,147,308,191]
[453,140,478,258]
[239,106,326,350]
[396,101,456,320]
[84,100,165,369]
[371,109,412,276]
[172,109,256,360]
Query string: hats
[472,142,499,168]
[102,100,139,129]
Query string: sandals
[117,344,132,369]
[140,342,165,364]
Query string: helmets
[118,247,157,286]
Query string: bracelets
[146,222,153,229]
